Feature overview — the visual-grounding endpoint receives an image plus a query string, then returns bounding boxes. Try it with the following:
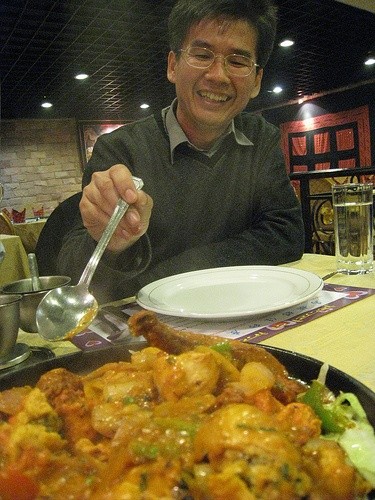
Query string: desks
[0,234,29,289]
[10,218,48,252]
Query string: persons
[36,0,305,307]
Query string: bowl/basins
[0,295,23,363]
[1,275,72,334]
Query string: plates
[135,265,324,322]
[0,339,375,499]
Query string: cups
[332,183,374,277]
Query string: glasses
[175,44,262,80]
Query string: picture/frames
[79,120,136,173]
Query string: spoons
[36,176,145,342]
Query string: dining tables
[16,252,375,391]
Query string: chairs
[0,212,15,235]
[290,167,375,256]
